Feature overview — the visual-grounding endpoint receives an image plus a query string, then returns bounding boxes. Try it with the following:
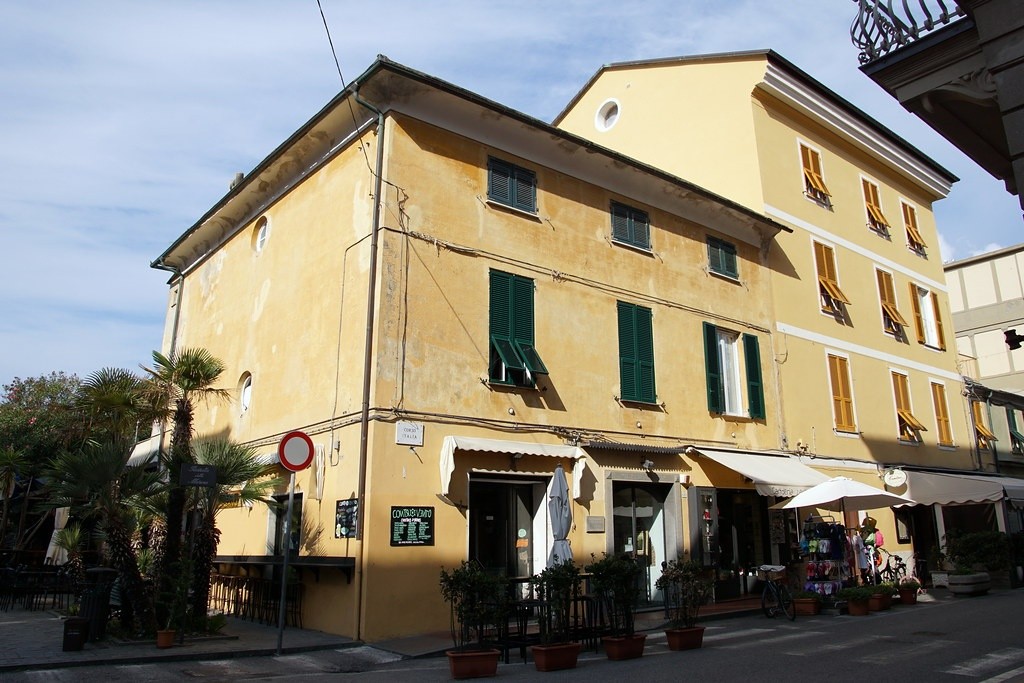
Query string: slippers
[799,522,845,595]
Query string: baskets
[758,565,785,581]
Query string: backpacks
[861,528,884,585]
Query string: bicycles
[878,547,908,590]
[753,566,796,622]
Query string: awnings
[439,434,588,499]
[888,469,1024,507]
[691,443,833,496]
[237,444,326,508]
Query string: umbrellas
[43,481,73,616]
[768,474,917,589]
[547,463,573,572]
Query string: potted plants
[588,551,652,661]
[439,557,512,679]
[894,579,923,605]
[156,584,186,649]
[784,592,824,616]
[864,585,898,612]
[947,566,992,599]
[838,588,873,615]
[530,558,585,673]
[928,546,952,589]
[655,559,718,652]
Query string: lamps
[643,460,653,473]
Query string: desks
[19,571,75,611]
[212,556,355,584]
[507,572,593,585]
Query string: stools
[0,563,70,612]
[472,592,626,665]
[210,572,304,630]
